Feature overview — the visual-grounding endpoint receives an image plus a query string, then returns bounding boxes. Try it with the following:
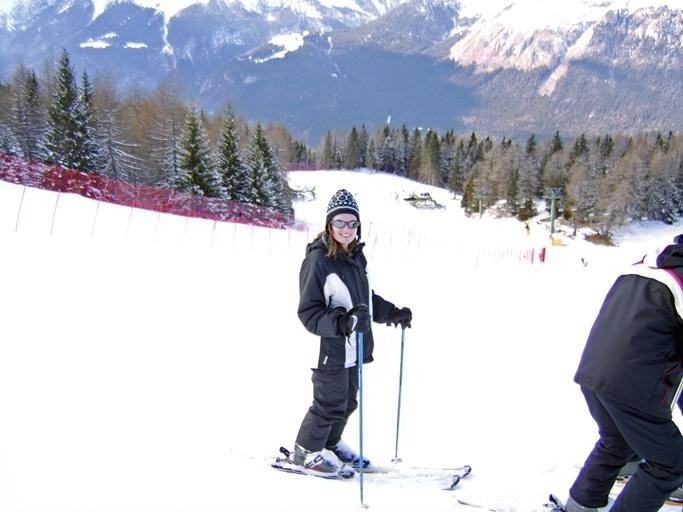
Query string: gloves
[339,302,370,335]
[388,305,412,329]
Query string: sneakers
[293,447,339,472]
[330,441,370,468]
[563,494,598,512]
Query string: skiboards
[268,447,472,491]
[458,499,547,512]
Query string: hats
[325,189,360,216]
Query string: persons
[608,454,682,504]
[289,187,413,474]
[564,232,683,512]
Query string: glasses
[330,220,359,229]
